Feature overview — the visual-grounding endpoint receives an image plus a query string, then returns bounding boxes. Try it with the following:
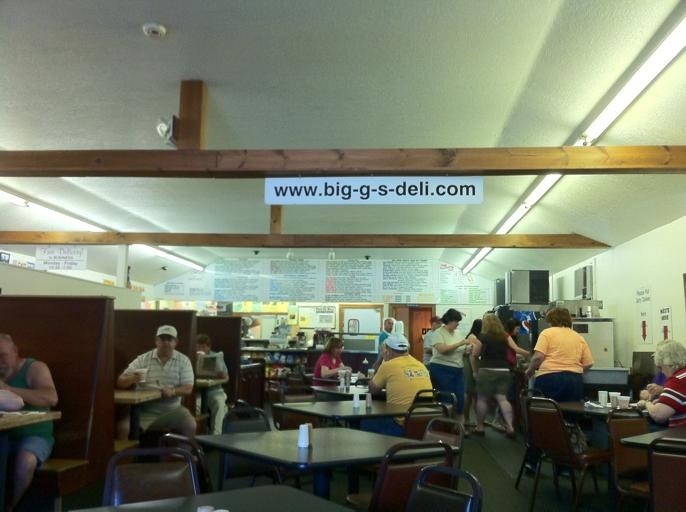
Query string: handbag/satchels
[565,422,590,454]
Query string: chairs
[104,363,686,512]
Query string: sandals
[455,426,469,438]
[506,426,516,438]
[471,427,486,437]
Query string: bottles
[362,356,369,377]
[297,448,313,466]
[297,423,313,448]
[365,407,372,415]
[353,407,359,415]
[352,392,360,408]
[366,393,372,407]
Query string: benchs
[0,293,268,510]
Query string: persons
[361,333,433,436]
[116,325,198,438]
[637,339,685,427]
[421,307,593,474]
[196,333,230,435]
[374,318,399,369]
[314,337,348,385]
[0,333,59,512]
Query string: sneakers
[491,420,506,432]
[524,462,537,473]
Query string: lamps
[463,18,686,274]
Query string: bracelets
[171,387,176,397]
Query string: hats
[155,325,177,339]
[385,333,409,351]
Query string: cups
[339,367,352,387]
[338,386,350,394]
[598,390,608,405]
[136,367,149,383]
[617,396,631,408]
[609,392,621,406]
[369,369,375,377]
